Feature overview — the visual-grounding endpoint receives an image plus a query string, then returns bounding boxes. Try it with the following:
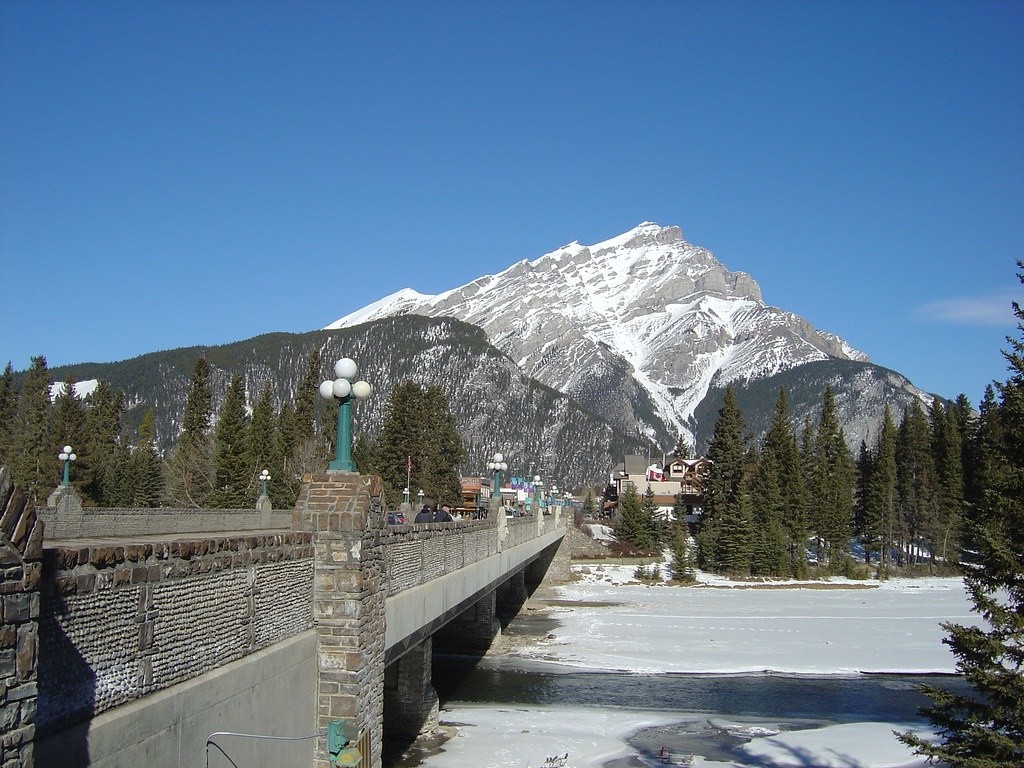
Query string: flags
[649,470,665,482]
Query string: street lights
[402,487,411,503]
[57,445,77,486]
[317,354,374,476]
[489,452,509,499]
[530,474,543,508]
[549,486,560,505]
[258,469,272,497]
[418,490,426,505]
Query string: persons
[414,504,478,522]
[519,510,528,517]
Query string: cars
[387,511,404,524]
